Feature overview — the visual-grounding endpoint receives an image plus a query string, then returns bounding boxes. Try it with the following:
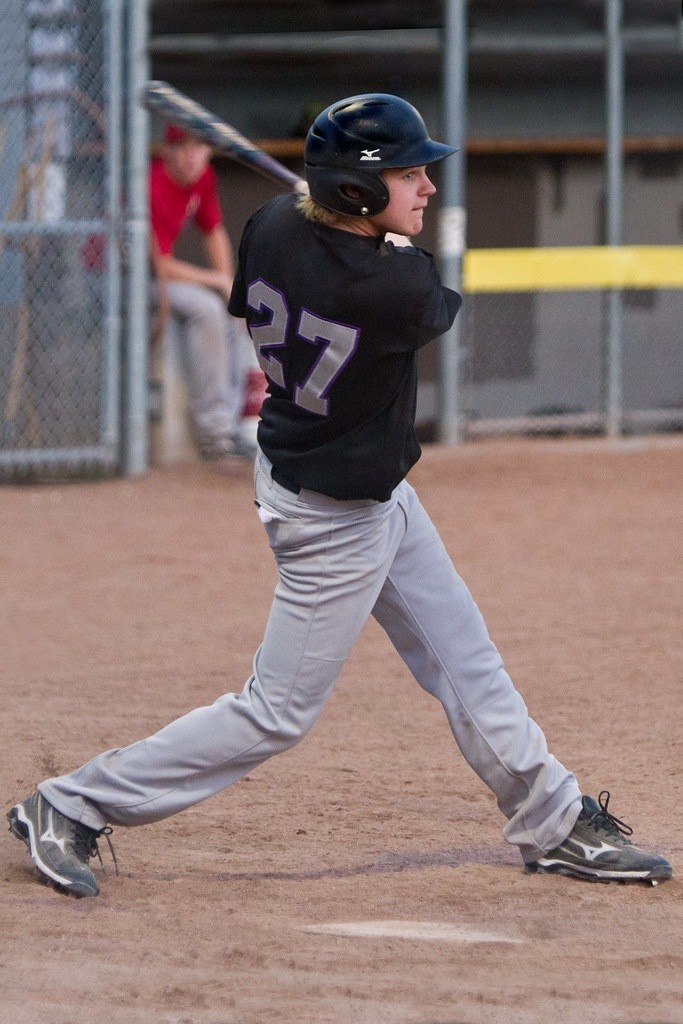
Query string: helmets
[303,92,458,218]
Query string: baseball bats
[138,81,411,248]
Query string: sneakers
[522,794,672,890]
[7,793,108,896]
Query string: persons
[82,110,271,464]
[0,88,672,908]
